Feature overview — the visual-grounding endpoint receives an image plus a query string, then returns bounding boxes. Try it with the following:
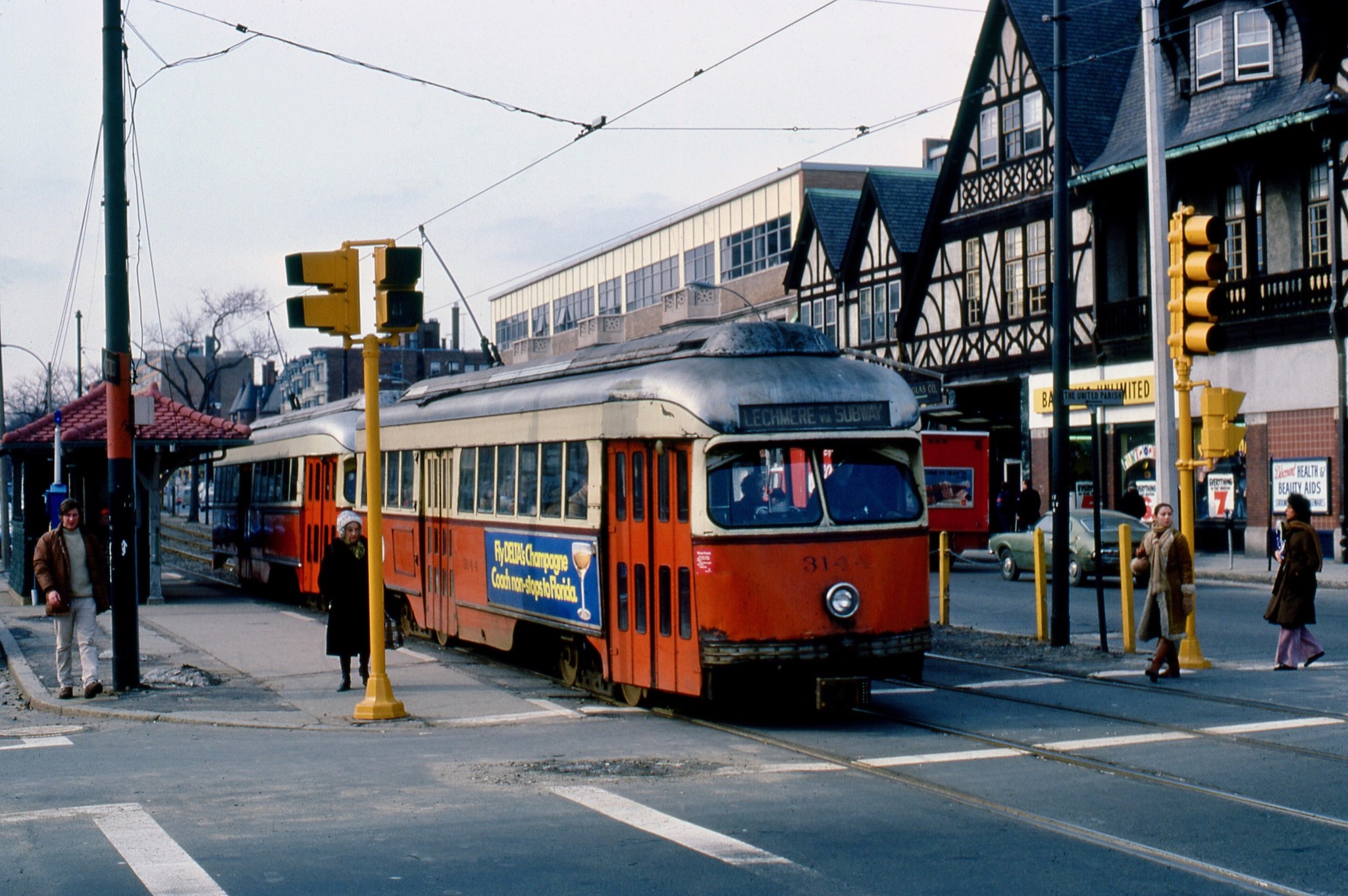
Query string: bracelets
[883,509,889,517]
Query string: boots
[1158,641,1180,679]
[1145,635,1174,683]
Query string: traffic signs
[1063,389,1124,406]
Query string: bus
[206,316,934,712]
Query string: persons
[997,482,1014,532]
[1017,479,1041,533]
[809,453,903,522]
[317,510,370,691]
[568,475,587,517]
[1262,493,1325,670]
[501,471,531,513]
[1132,503,1196,683]
[33,498,113,699]
[732,474,770,521]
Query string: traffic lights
[1199,385,1250,458]
[373,245,423,332]
[1167,203,1234,360]
[284,247,361,337]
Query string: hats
[335,509,363,538]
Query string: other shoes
[59,687,72,698]
[1272,662,1297,671]
[84,683,102,699]
[1304,650,1325,668]
[358,666,370,686]
[336,673,350,692]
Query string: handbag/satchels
[383,610,404,650]
[1135,547,1151,585]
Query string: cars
[988,507,1153,589]
